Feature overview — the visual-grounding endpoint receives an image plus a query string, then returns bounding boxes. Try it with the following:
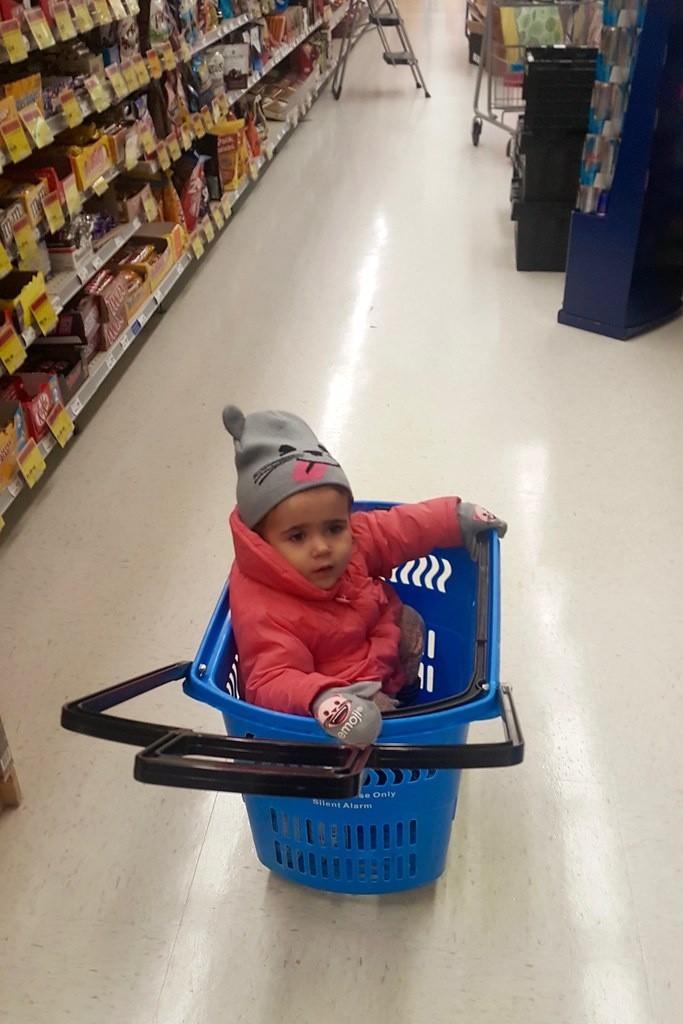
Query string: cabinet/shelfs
[0,0,386,546]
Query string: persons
[220,402,509,864]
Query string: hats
[223,404,354,530]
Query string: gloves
[310,681,383,750]
[456,502,507,562]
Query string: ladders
[331,0,431,101]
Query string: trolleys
[471,0,581,164]
